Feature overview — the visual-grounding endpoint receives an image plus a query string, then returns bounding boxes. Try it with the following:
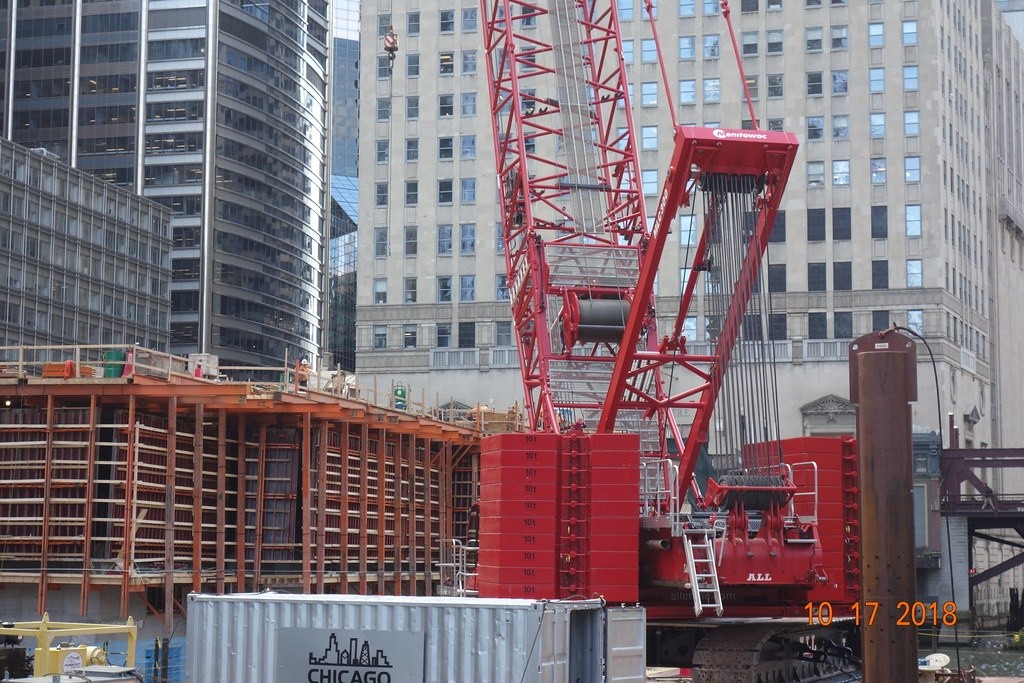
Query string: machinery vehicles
[384,0,862,683]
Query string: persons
[296,359,310,392]
[981,483,996,510]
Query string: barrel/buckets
[104,352,124,378]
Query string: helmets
[301,359,307,365]
